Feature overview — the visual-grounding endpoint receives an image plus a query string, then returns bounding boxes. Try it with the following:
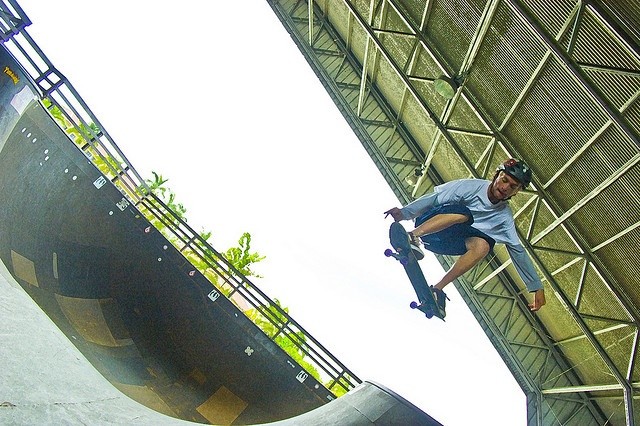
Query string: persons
[383,158,546,318]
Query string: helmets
[495,157,533,187]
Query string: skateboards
[384,222,446,322]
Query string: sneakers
[429,285,450,318]
[406,232,424,260]
